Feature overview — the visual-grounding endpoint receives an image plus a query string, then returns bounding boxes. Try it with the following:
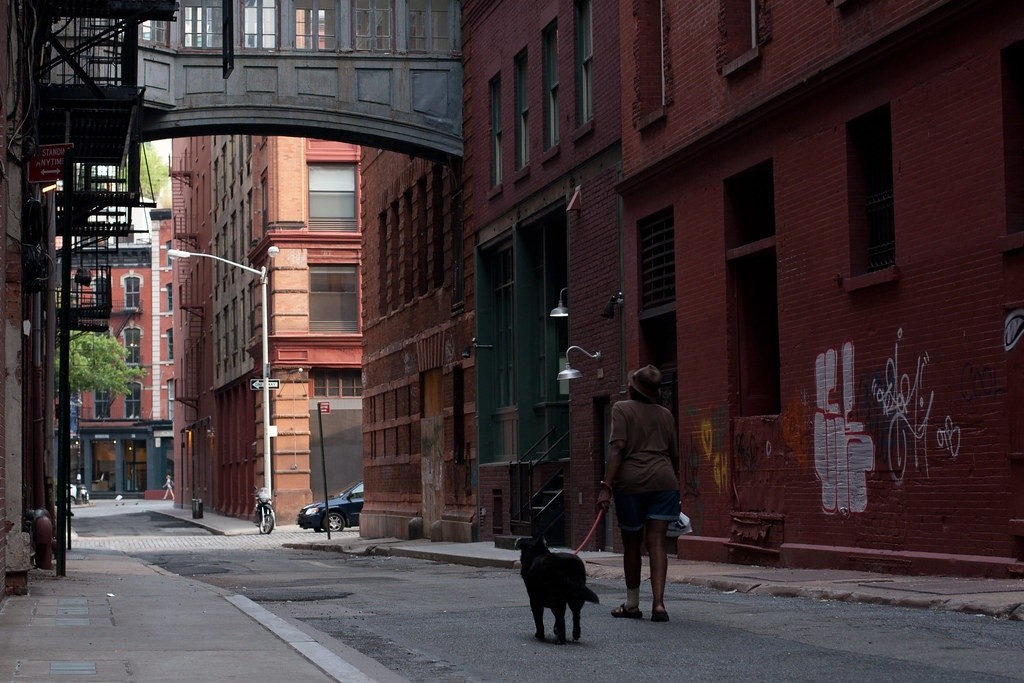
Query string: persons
[162,474,175,502]
[597,365,682,622]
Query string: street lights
[168,249,272,515]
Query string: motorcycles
[251,485,277,535]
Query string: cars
[78,480,108,492]
[66,484,89,504]
[295,479,365,532]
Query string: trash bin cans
[75,486,86,504]
[191,498,204,519]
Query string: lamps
[557,345,601,380]
[603,292,623,319]
[550,287,569,317]
[461,345,473,358]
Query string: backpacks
[170,479,174,486]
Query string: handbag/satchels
[668,513,693,540]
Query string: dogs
[513,529,601,646]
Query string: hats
[627,364,664,403]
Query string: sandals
[612,604,642,619]
[652,608,670,622]
